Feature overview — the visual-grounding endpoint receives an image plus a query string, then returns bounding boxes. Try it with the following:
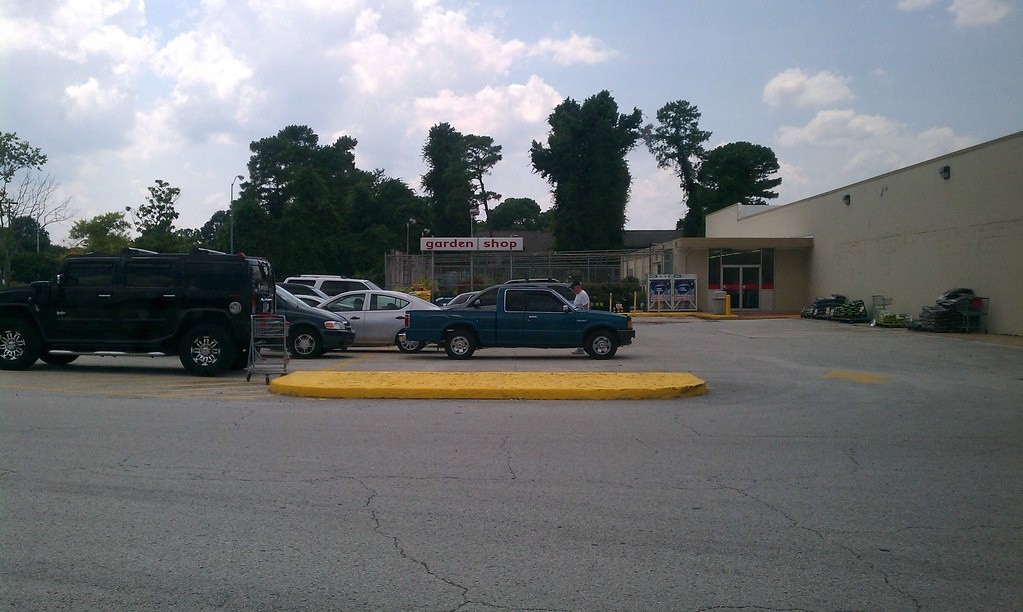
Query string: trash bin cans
[712,291,727,315]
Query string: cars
[442,290,483,306]
[272,282,356,360]
[313,290,444,354]
[274,274,382,312]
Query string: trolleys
[871,294,895,327]
[243,311,291,384]
[954,294,990,334]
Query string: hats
[569,280,582,288]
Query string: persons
[569,282,590,354]
[353,299,362,309]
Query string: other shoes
[572,348,584,354]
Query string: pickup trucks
[404,287,637,359]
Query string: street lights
[229,175,245,253]
[406,218,417,288]
[467,208,482,293]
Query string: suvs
[440,278,575,308]
[0,245,276,378]
